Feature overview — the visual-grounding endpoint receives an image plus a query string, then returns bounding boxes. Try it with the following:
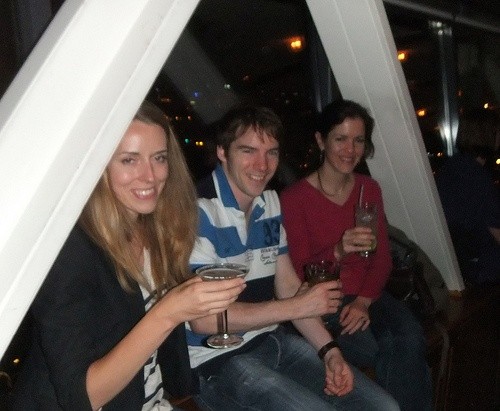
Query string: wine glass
[303,258,338,324]
[354,201,377,257]
[196,262,244,349]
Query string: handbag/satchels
[385,223,451,323]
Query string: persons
[16,100,246,411]
[278,100,432,411]
[185,103,401,411]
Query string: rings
[361,317,366,322]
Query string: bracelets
[318,341,340,359]
[337,239,353,257]
